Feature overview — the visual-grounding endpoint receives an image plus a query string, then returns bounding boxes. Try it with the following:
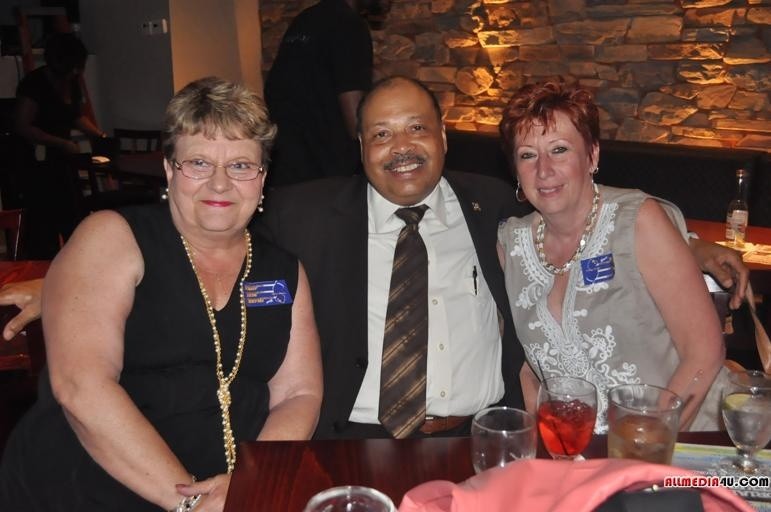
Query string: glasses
[172,158,263,181]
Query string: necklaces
[177,228,252,474]
[537,181,600,275]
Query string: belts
[421,415,466,433]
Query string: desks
[218,430,771,512]
[71,147,167,192]
[1,259,55,370]
[681,215,771,278]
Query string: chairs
[0,204,23,263]
[704,269,766,381]
[0,302,46,401]
[112,127,161,153]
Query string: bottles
[723,168,750,248]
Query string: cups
[304,481,395,512]
[466,406,537,478]
[536,375,594,459]
[602,382,684,467]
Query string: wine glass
[715,369,770,479]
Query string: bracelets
[169,472,203,511]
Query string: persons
[0,76,326,512]
[263,1,392,184]
[0,76,756,439]
[496,80,746,431]
[13,32,107,262]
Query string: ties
[377,205,430,439]
